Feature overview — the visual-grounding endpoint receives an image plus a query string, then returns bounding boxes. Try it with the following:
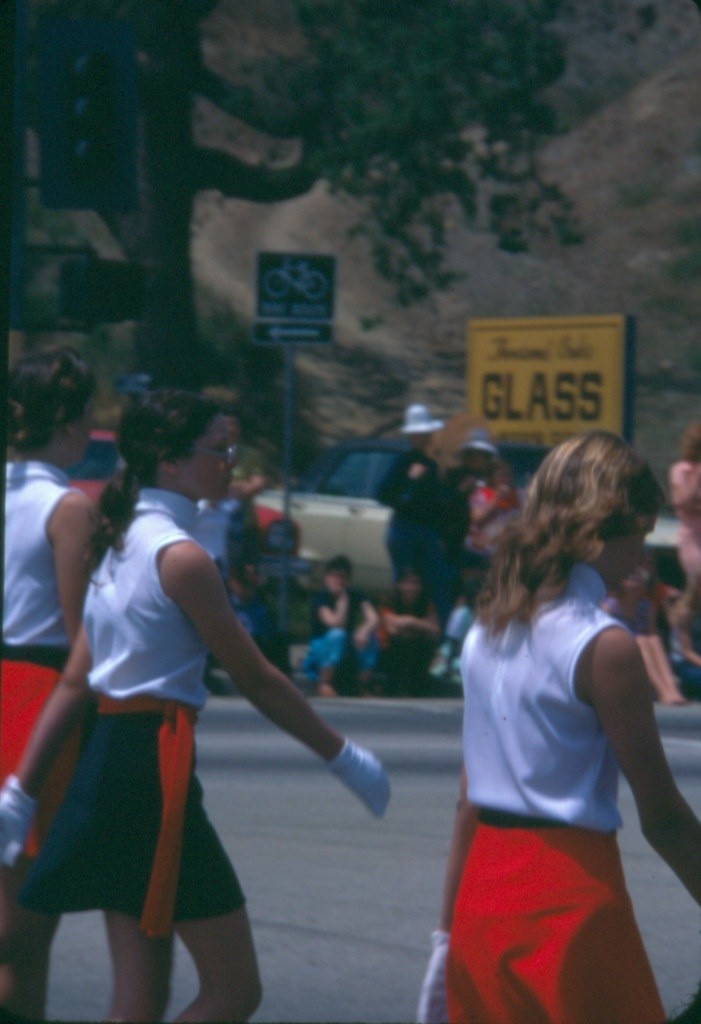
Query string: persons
[600,424,700,706]
[1,345,103,1024]
[0,389,390,1024]
[204,388,519,697]
[414,432,701,1024]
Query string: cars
[250,437,554,620]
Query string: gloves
[416,928,449,1024]
[0,775,38,867]
[327,735,392,818]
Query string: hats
[398,404,444,434]
[457,428,497,454]
